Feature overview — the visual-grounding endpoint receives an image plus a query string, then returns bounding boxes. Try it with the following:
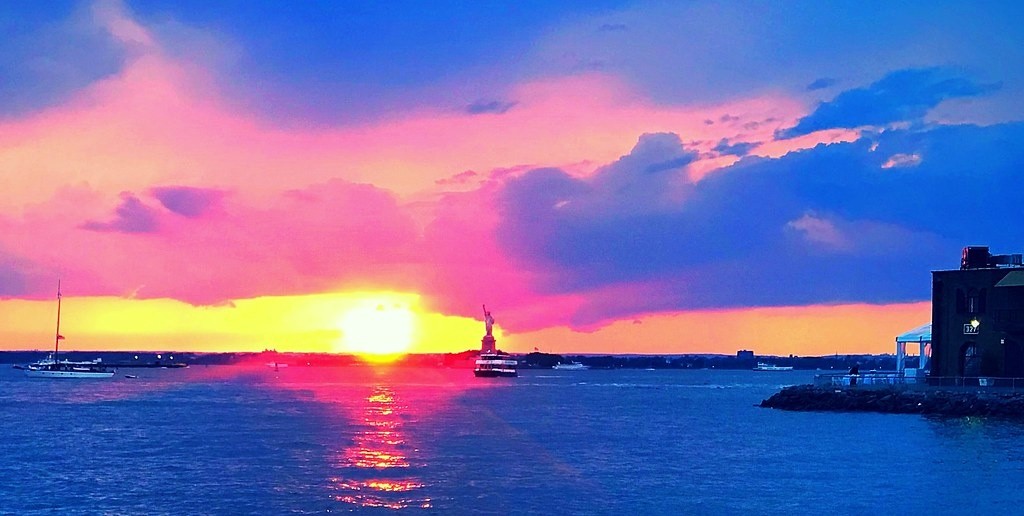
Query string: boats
[473,354,519,377]
[754,362,793,371]
[552,361,590,371]
[13,279,116,378]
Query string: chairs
[831,368,918,387]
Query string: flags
[535,347,539,351]
[56,335,65,340]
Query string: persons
[483,304,495,337]
[849,364,860,385]
